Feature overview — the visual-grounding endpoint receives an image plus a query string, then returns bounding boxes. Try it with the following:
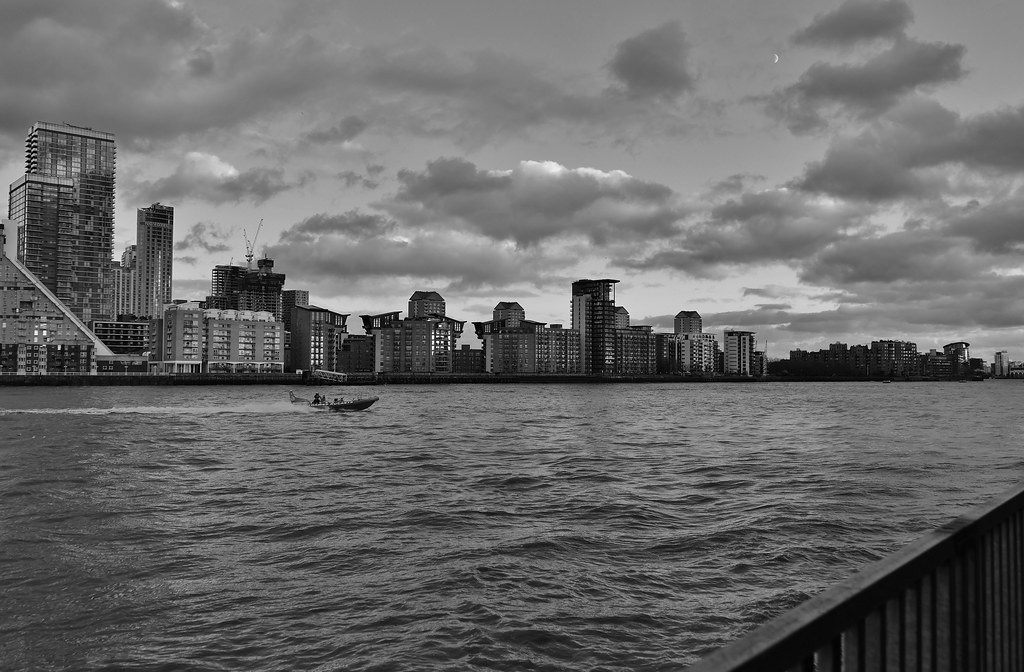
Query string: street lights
[64,365,67,372]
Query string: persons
[312,393,326,403]
[339,398,344,403]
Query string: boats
[289,390,379,413]
[883,380,890,383]
